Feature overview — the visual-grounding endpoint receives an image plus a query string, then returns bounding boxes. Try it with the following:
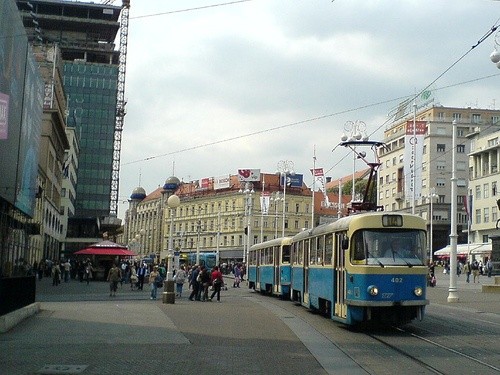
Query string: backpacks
[196,271,203,282]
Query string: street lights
[125,229,146,268]
[424,186,439,275]
[491,28,500,71]
[273,159,296,237]
[163,195,181,304]
[340,118,370,209]
[239,182,255,263]
[270,190,285,238]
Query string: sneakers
[195,298,201,301]
[189,296,193,301]
[233,285,236,288]
[237,286,240,288]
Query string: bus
[246,210,431,328]
[187,253,216,273]
[165,253,187,270]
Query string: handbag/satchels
[214,278,221,287]
[234,277,240,284]
[131,274,137,280]
[154,275,163,288]
[131,278,139,284]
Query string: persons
[150,266,160,300]
[121,258,149,292]
[209,266,223,302]
[218,260,256,287]
[430,256,495,283]
[22,256,95,286]
[173,265,186,296]
[107,261,122,297]
[187,261,210,302]
